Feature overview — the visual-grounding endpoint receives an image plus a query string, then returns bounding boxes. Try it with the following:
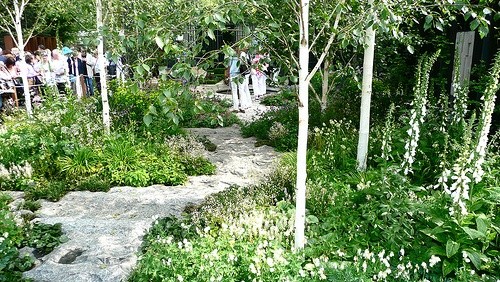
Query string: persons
[229,44,253,113]
[241,42,251,65]
[251,45,271,100]
[0,44,127,119]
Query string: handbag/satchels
[35,76,43,84]
[15,84,24,95]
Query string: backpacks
[240,58,251,75]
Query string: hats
[41,50,48,55]
[61,47,73,55]
